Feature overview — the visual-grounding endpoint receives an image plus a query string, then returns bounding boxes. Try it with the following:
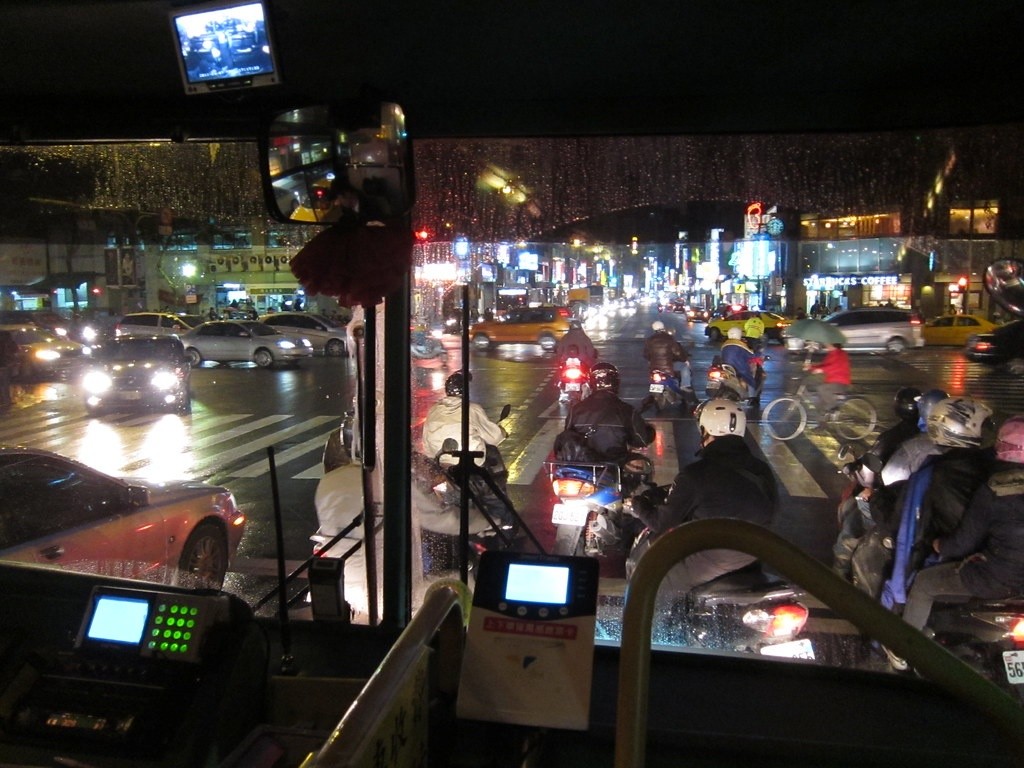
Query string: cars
[705,309,796,345]
[178,319,314,370]
[0,444,245,595]
[920,312,1002,347]
[274,178,358,222]
[255,310,349,358]
[82,335,194,416]
[113,312,195,338]
[469,303,573,353]
[0,324,93,382]
[563,287,750,323]
[962,317,1024,376]
[686,305,709,324]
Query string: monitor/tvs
[168,0,282,96]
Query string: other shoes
[882,643,907,671]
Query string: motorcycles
[611,438,1024,708]
[543,395,656,558]
[302,404,513,625]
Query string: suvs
[1,308,107,349]
[820,305,926,355]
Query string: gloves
[632,494,649,513]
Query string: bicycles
[761,369,879,442]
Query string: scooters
[705,348,773,412]
[649,340,698,413]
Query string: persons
[554,320,598,368]
[830,384,1024,673]
[153,306,171,313]
[230,299,238,307]
[314,410,504,621]
[885,298,893,307]
[331,133,403,214]
[721,327,760,389]
[801,341,851,418]
[743,310,764,349]
[563,361,655,498]
[622,396,776,592]
[293,299,303,311]
[643,320,694,393]
[281,301,289,311]
[207,307,217,321]
[267,307,274,314]
[422,369,508,468]
[795,299,831,319]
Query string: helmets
[693,398,747,438]
[896,386,922,422]
[652,321,664,331]
[915,389,949,432]
[444,369,472,397]
[728,328,741,340]
[571,321,581,330]
[927,395,996,452]
[989,415,1024,463]
[340,415,360,457]
[590,361,619,394]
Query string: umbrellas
[783,318,847,344]
